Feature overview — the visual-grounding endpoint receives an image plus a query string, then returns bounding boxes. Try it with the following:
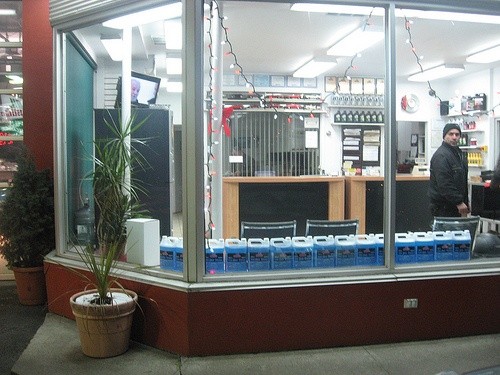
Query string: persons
[230,145,255,177]
[429,123,470,217]
[131,79,140,103]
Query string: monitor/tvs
[115,71,161,109]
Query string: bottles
[467,153,482,165]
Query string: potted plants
[70,104,155,359]
[0,142,54,305]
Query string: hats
[443,123,461,142]
